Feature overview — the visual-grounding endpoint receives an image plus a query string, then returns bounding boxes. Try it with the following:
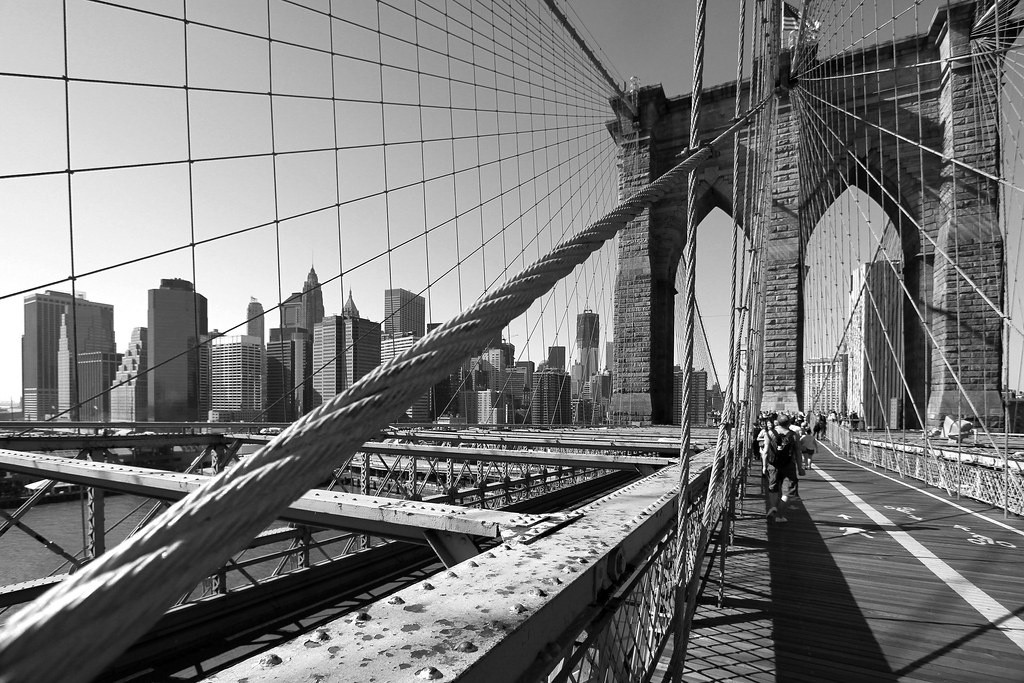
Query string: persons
[827,409,860,439]
[709,407,721,426]
[799,429,818,470]
[761,415,805,523]
[756,419,775,475]
[790,409,826,441]
[750,409,790,460]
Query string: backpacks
[766,429,795,464]
[819,421,824,428]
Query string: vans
[260,428,280,435]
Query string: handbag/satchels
[800,445,806,451]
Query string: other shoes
[766,506,777,520]
[805,466,811,470]
[775,517,788,522]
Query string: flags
[784,2,816,31]
